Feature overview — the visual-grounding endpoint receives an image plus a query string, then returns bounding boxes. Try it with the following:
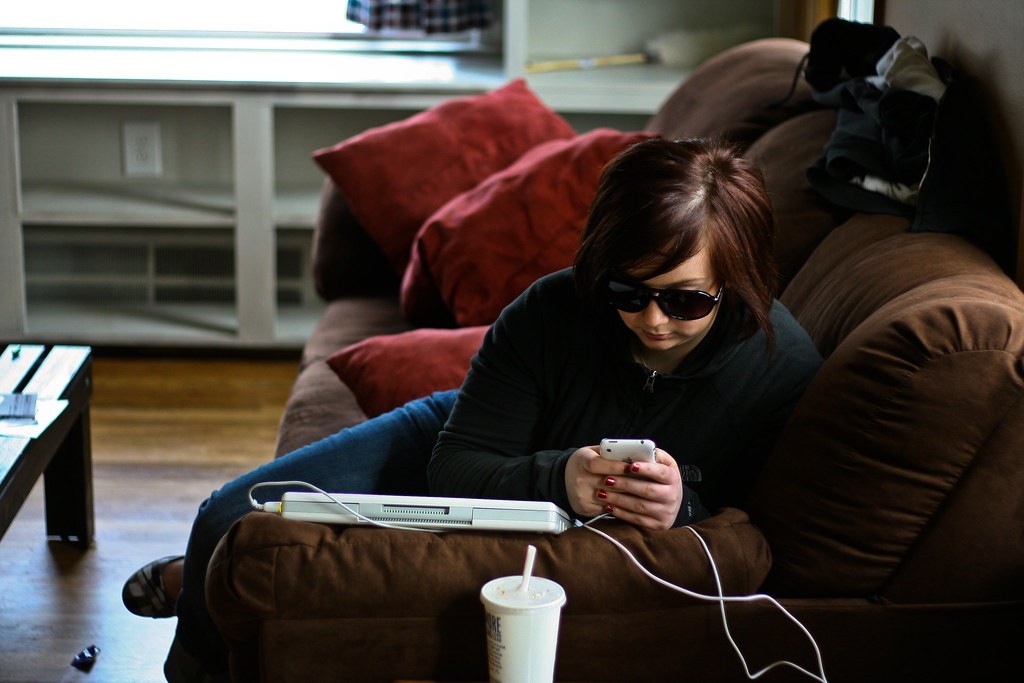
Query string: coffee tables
[0,340,100,549]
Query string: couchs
[166,22,1021,682]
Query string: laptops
[280,491,572,534]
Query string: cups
[480,574,567,683]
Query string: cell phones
[598,439,658,480]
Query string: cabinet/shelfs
[0,30,724,347]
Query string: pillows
[324,324,500,420]
[320,78,582,269]
[397,125,674,320]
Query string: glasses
[605,272,725,323]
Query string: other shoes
[121,554,183,619]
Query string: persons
[118,136,826,683]
